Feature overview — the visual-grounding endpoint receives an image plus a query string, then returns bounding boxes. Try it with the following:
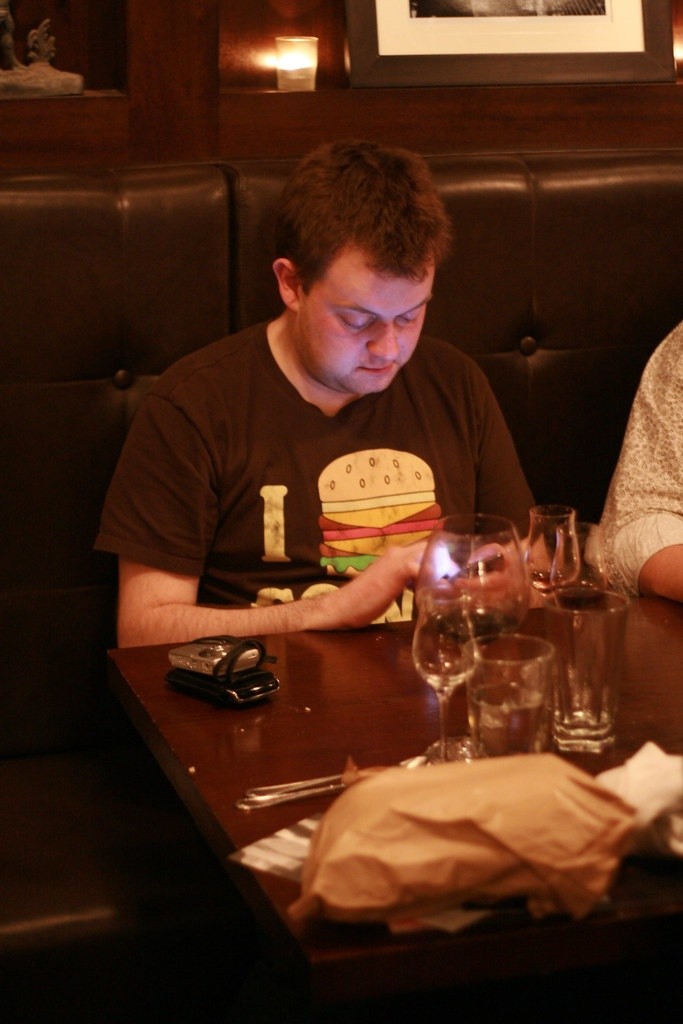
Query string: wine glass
[413,514,532,764]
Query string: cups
[526,507,580,599]
[463,634,554,757]
[551,522,607,610]
[546,590,629,752]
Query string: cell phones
[448,552,504,583]
[166,667,279,705]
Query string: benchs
[0,145,682,957]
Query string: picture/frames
[345,0,675,89]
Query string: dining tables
[102,598,683,1024]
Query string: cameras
[168,640,261,678]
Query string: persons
[92,139,554,652]
[594,321,682,600]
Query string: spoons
[236,738,484,809]
